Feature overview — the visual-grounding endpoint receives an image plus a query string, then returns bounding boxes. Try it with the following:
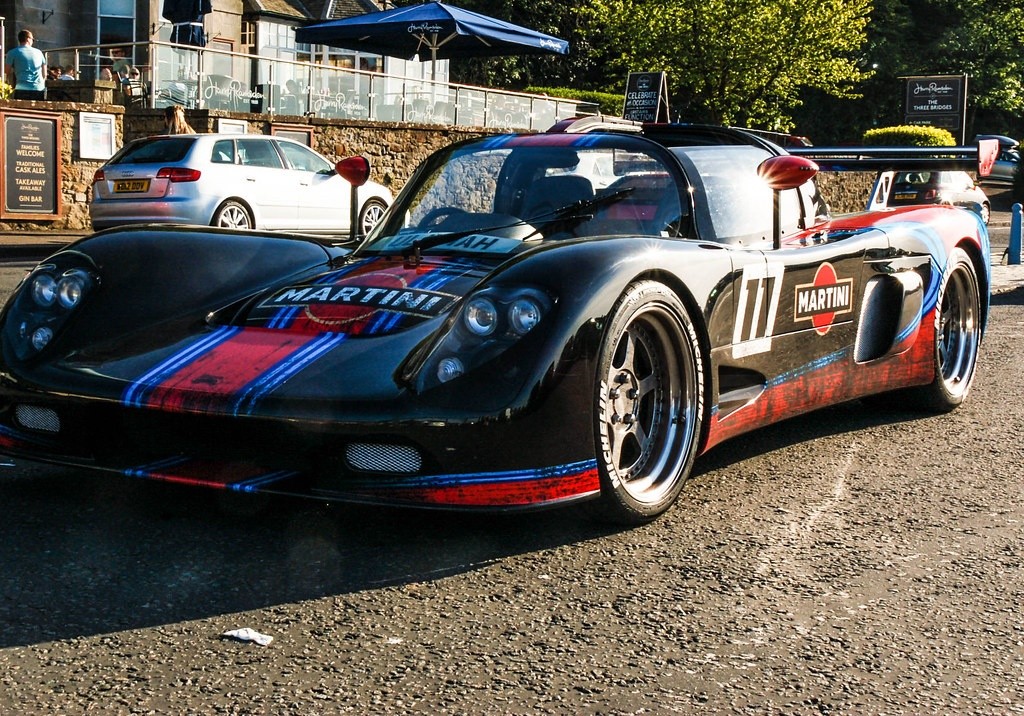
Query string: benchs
[46,80,116,104]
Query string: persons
[286,79,296,93]
[4,30,47,101]
[536,92,554,114]
[100,49,144,107]
[50,65,75,80]
[492,94,531,126]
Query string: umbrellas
[291,0,568,107]
[162,0,212,80]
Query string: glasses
[131,72,138,76]
[26,37,34,42]
[112,51,122,54]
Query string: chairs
[263,84,297,116]
[206,74,234,110]
[521,175,593,221]
[411,98,456,122]
[325,90,356,118]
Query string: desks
[162,80,205,108]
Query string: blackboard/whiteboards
[623,70,671,124]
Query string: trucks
[729,126,820,168]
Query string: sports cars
[0,113,995,530]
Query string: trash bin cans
[248,84,282,115]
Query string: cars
[888,170,992,227]
[963,133,1021,182]
[86,131,411,247]
